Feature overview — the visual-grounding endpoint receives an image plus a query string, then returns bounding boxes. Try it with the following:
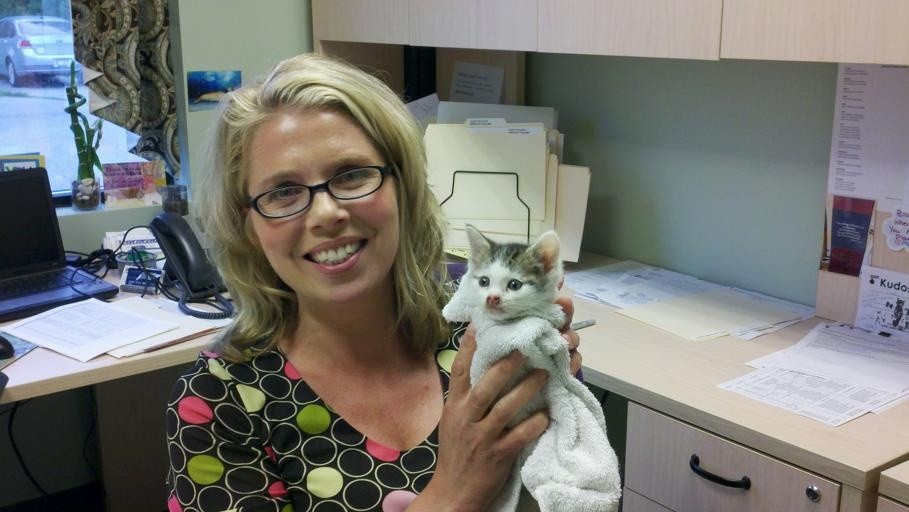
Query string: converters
[65,256,81,266]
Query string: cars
[0,16,81,87]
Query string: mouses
[0,336,15,360]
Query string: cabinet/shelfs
[720,1,909,68]
[539,1,724,62]
[313,0,412,46]
[409,1,539,53]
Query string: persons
[164,53,582,512]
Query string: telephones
[149,213,228,300]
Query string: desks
[0,263,232,511]
[556,249,908,511]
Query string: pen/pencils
[144,326,217,352]
[570,320,595,331]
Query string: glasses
[245,163,391,220]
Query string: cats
[464,223,565,512]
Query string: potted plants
[64,59,108,213]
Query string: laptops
[0,168,119,322]
